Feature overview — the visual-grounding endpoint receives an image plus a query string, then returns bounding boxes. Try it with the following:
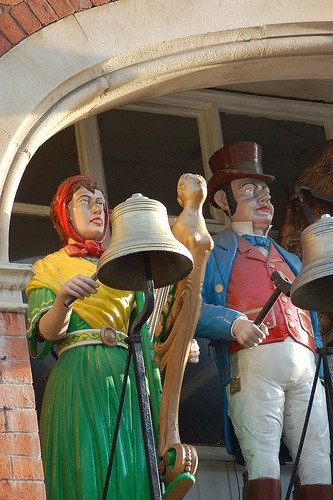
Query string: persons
[197,140,333,500]
[25,174,201,500]
